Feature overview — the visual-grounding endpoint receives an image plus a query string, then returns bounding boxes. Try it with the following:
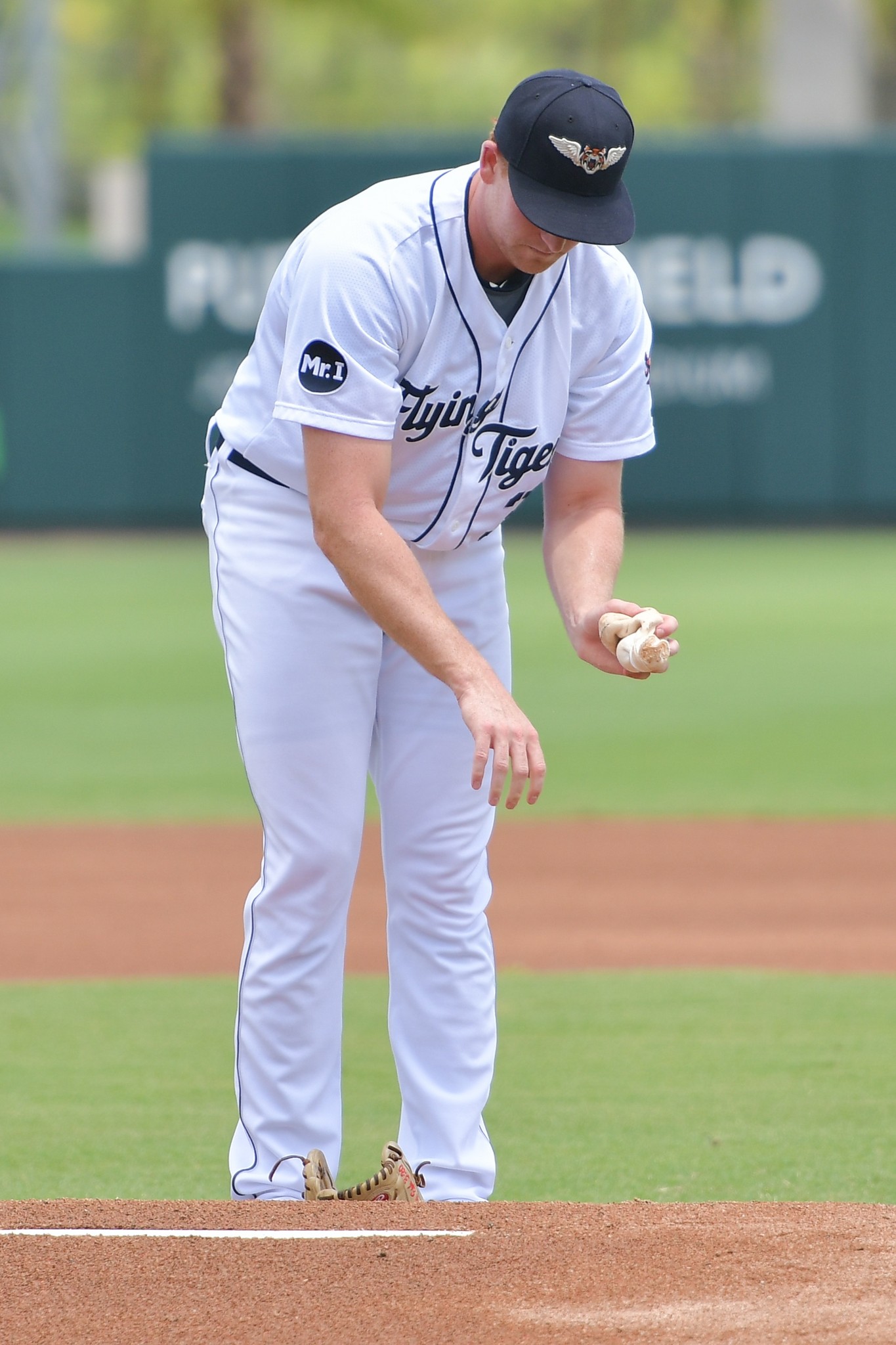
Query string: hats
[494,69,635,246]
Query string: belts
[208,423,289,489]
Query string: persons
[200,68,679,1202]
